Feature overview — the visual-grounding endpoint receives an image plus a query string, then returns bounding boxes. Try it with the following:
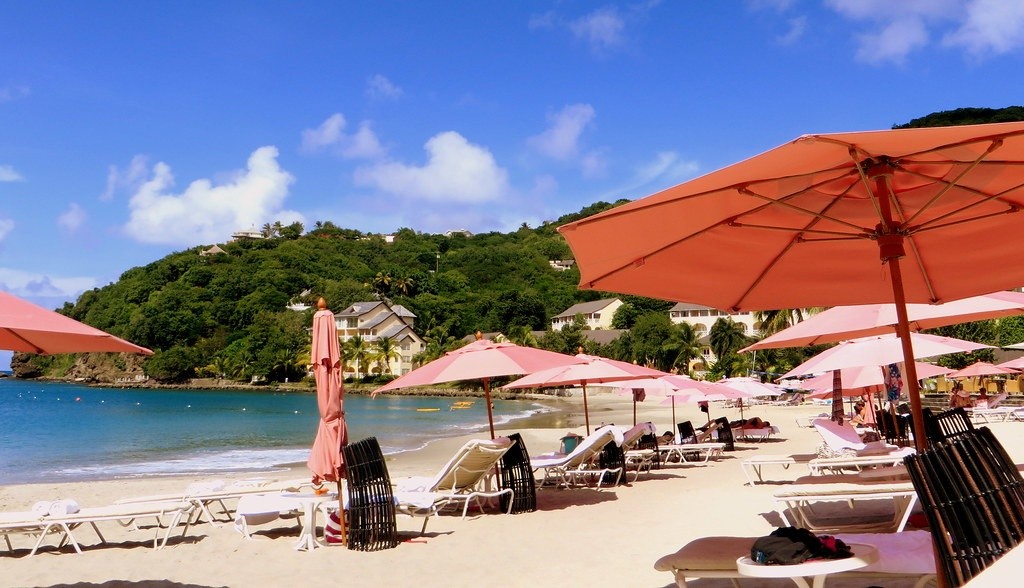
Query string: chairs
[0,393,1024,588]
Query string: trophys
[656,431,674,443]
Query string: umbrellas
[307,297,350,546]
[0,288,155,355]
[372,122,1024,588]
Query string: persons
[694,419,724,441]
[729,417,770,429]
[490,402,494,409]
[854,400,865,416]
[949,384,989,407]
